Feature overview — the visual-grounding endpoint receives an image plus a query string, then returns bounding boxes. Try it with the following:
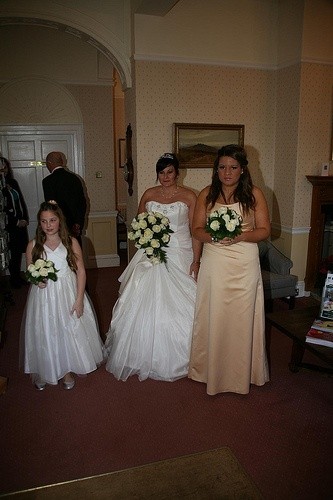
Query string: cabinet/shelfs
[303,174,333,300]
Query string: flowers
[206,206,244,243]
[127,211,174,263]
[27,258,62,284]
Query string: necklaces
[157,183,180,199]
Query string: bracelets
[191,260,200,264]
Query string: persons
[103,153,205,383]
[40,152,89,253]
[187,143,272,395]
[18,200,107,391]
[0,152,30,344]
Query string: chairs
[258,240,300,309]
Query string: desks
[0,446,269,500]
[265,304,333,382]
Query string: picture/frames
[119,139,127,168]
[172,122,245,169]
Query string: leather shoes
[62,377,75,390]
[33,374,47,391]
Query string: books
[304,273,333,346]
